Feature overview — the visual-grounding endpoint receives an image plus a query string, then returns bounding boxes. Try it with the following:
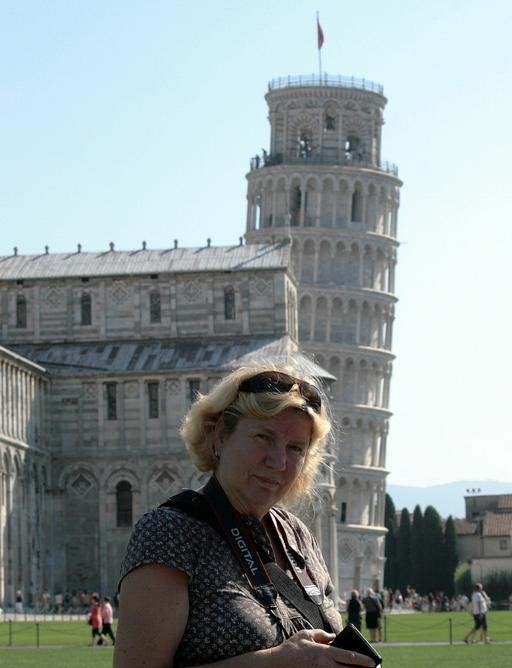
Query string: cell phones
[329,623,382,668]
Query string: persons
[347,588,363,631]
[363,588,382,644]
[86,595,109,647]
[13,587,120,616]
[346,583,493,614]
[109,363,379,667]
[469,588,491,642]
[98,597,117,646]
[462,583,491,645]
[374,590,383,641]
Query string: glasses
[238,369,325,413]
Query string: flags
[318,25,324,49]
